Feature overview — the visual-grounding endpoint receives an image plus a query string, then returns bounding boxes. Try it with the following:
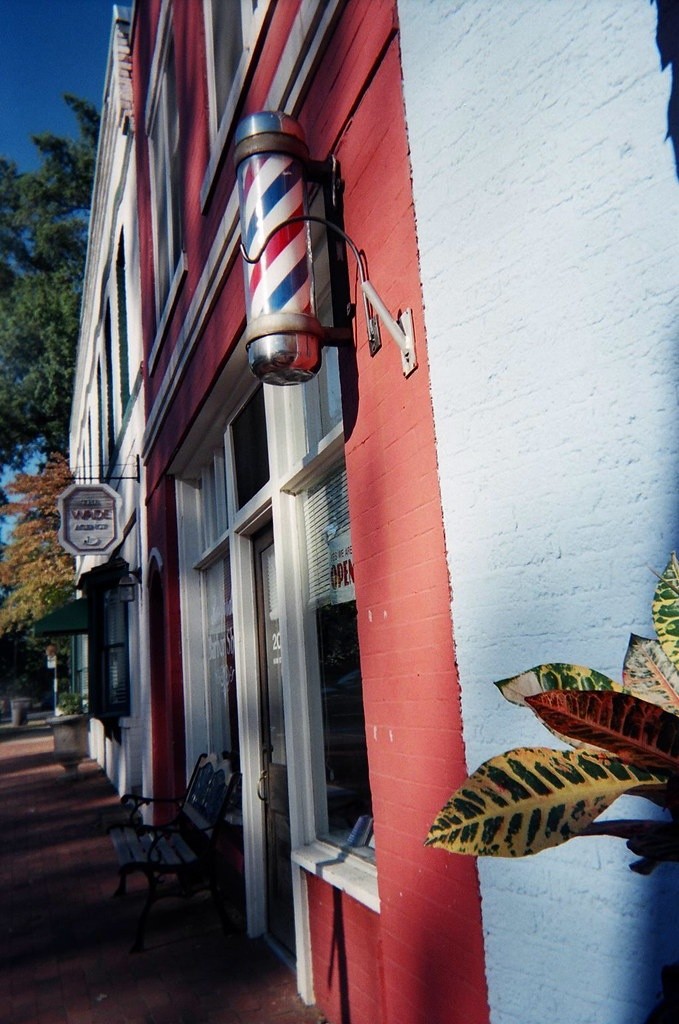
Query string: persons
[36,644,68,710]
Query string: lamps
[118,567,142,602]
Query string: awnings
[33,598,88,636]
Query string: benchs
[105,752,241,956]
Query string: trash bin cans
[11,698,31,727]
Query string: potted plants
[46,691,91,791]
